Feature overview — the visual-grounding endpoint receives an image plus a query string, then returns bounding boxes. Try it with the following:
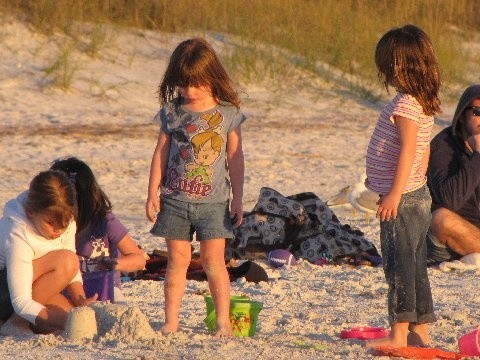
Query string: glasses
[467,106,480,116]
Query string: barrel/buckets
[82,269,123,302]
[204,294,264,337]
[458,328,480,356]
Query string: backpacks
[228,187,381,266]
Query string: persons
[426,82,480,266]
[145,37,248,339]
[1,169,98,339]
[51,159,146,309]
[364,24,443,352]
